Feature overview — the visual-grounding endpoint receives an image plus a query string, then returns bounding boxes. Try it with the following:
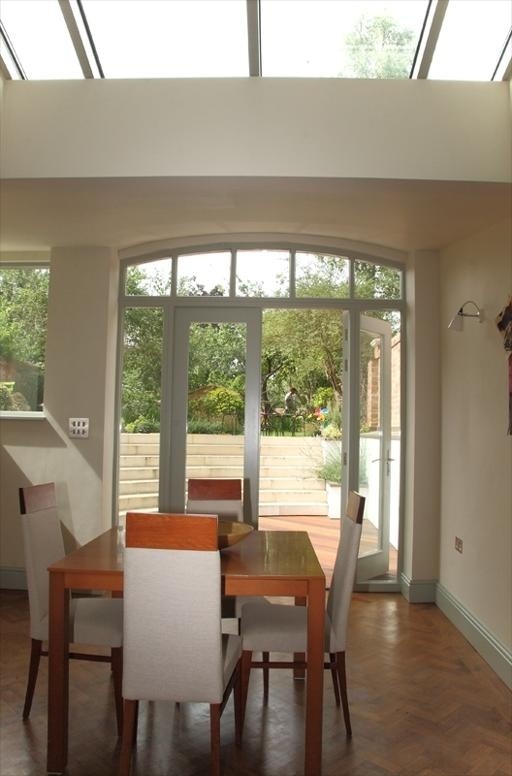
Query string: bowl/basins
[218,520,255,551]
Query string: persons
[284,388,302,422]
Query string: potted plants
[301,438,369,519]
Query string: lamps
[447,299,485,332]
